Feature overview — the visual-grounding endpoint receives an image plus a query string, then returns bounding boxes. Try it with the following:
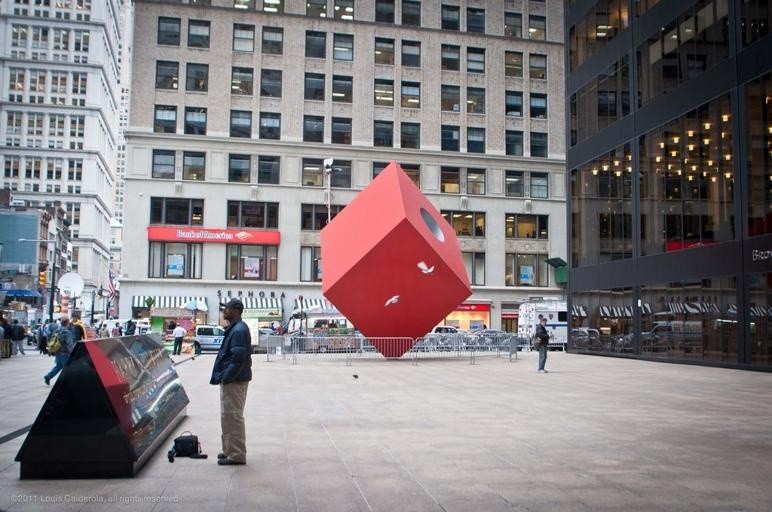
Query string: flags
[107,271,116,303]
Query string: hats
[219,298,244,313]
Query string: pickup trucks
[166,323,227,352]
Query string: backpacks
[532,336,542,350]
[45,329,62,354]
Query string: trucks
[95,319,150,336]
[518,298,568,351]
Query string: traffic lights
[55,305,61,313]
[40,271,46,286]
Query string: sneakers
[538,369,547,373]
[12,349,58,357]
[43,375,51,385]
[172,352,181,355]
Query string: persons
[534,318,551,373]
[0,313,190,385]
[212,298,254,466]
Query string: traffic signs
[47,288,60,292]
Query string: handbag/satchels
[168,430,207,463]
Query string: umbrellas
[180,300,208,323]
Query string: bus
[288,315,356,352]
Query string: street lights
[90,289,96,324]
[299,295,304,336]
[18,238,58,321]
[322,159,344,222]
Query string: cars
[412,325,523,351]
[259,329,275,336]
[572,321,703,355]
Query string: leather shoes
[217,453,246,465]
